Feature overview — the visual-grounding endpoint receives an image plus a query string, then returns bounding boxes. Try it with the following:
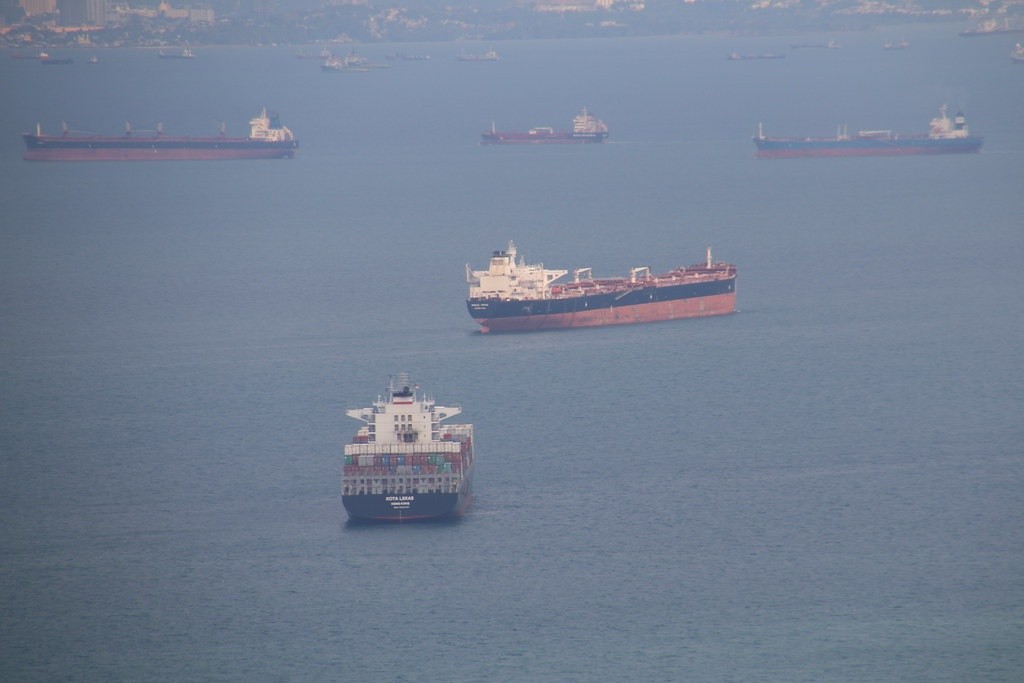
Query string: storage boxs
[343,430,469,493]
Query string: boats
[340,372,476,524]
[463,240,741,335]
[457,49,499,61]
[20,104,302,163]
[158,48,196,59]
[479,104,610,144]
[288,45,432,73]
[10,44,99,66]
[755,101,985,161]
